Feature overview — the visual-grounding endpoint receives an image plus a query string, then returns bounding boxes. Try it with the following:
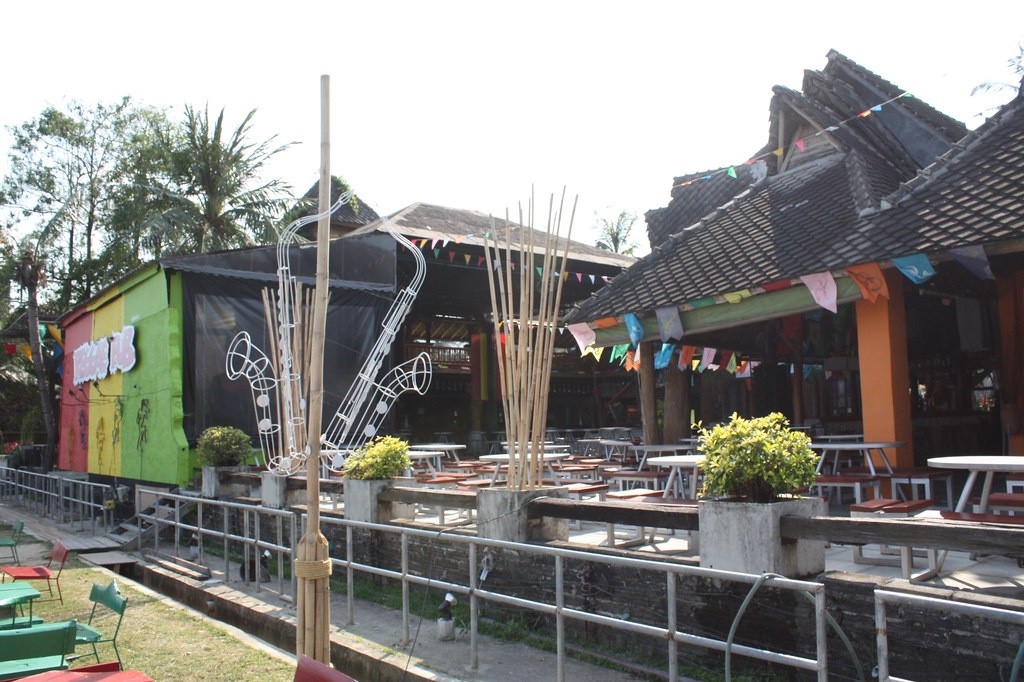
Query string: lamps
[188,534,197,546]
[437,592,457,620]
[258,548,271,566]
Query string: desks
[320,427,907,544]
[0,581,41,629]
[926,452,1023,572]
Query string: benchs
[320,438,1024,567]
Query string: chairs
[0,519,127,678]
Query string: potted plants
[341,435,414,523]
[690,412,824,577]
[195,425,253,498]
